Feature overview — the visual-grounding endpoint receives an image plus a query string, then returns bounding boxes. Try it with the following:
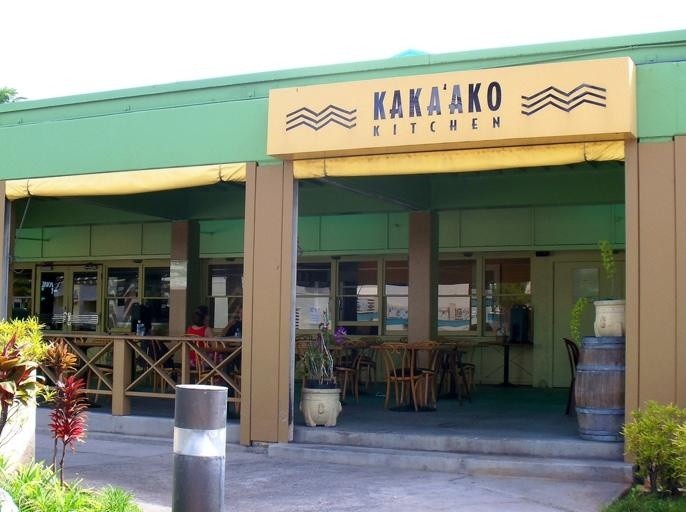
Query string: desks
[477,341,534,388]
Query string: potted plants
[565,237,625,336]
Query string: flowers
[294,309,349,387]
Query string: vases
[298,387,343,426]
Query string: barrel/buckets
[573,336,625,443]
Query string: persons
[219,303,241,375]
[186,303,227,387]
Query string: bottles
[136,319,145,336]
[235,327,241,337]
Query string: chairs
[54,329,240,414]
[293,334,478,412]
[559,335,579,415]
